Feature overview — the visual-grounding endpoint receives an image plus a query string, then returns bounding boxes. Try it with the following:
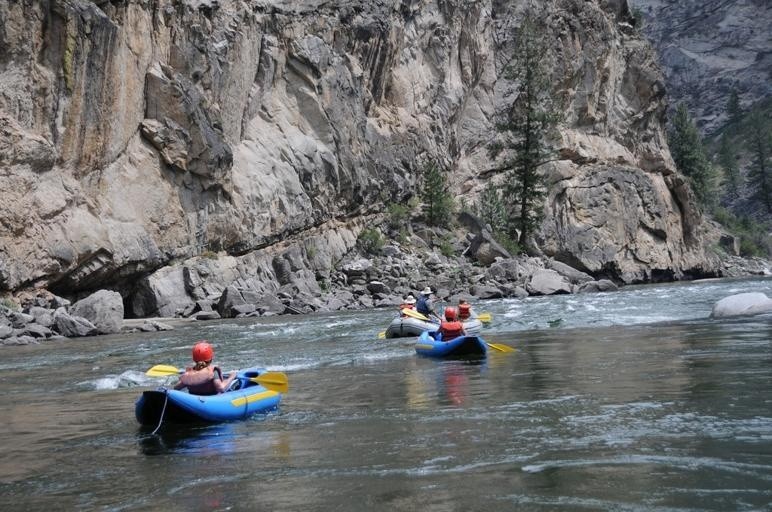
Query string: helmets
[193,342,213,362]
[445,306,456,319]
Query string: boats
[384,308,484,339]
[413,328,488,360]
[134,367,282,423]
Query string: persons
[399,294,417,320]
[456,298,473,321]
[434,305,468,341]
[172,342,242,395]
[415,286,443,321]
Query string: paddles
[403,307,515,354]
[146,365,289,394]
[378,314,490,340]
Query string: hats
[405,295,417,304]
[420,286,433,295]
